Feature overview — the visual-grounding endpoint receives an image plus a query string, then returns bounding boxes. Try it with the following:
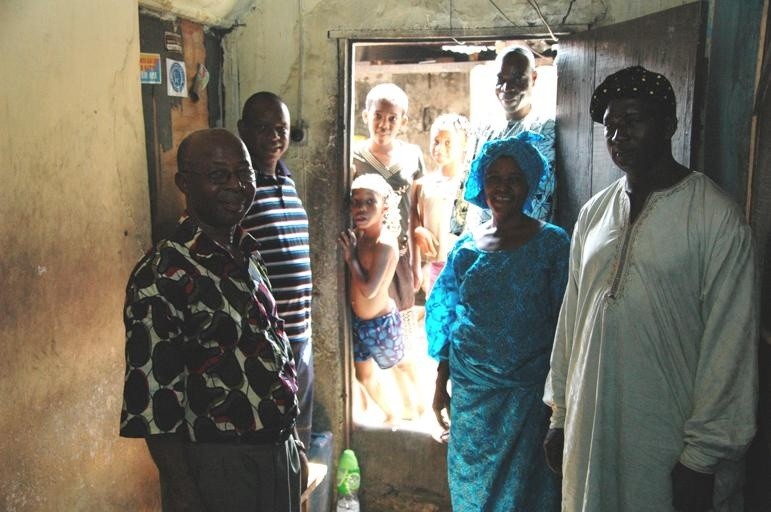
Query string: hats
[590,66,676,124]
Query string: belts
[207,425,294,444]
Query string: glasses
[182,167,259,183]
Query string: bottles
[334,449,360,512]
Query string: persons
[449,47,555,236]
[354,83,424,419]
[425,131,569,512]
[236,87,309,455]
[545,65,761,512]
[336,173,424,425]
[116,128,309,511]
[411,113,475,289]
